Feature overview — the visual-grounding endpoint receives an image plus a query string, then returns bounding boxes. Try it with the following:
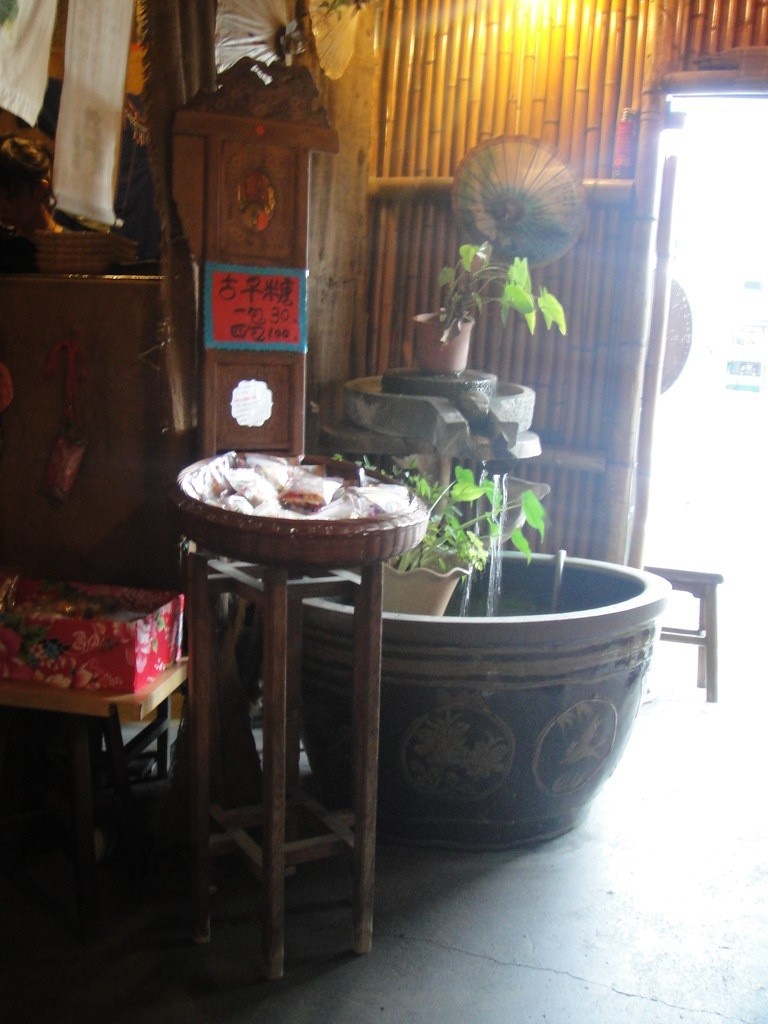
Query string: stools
[644,566,723,703]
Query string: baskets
[172,450,429,559]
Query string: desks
[185,550,381,981]
[0,272,191,588]
[0,656,190,949]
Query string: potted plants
[412,242,567,375]
[334,455,549,614]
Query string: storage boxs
[0,571,185,696]
